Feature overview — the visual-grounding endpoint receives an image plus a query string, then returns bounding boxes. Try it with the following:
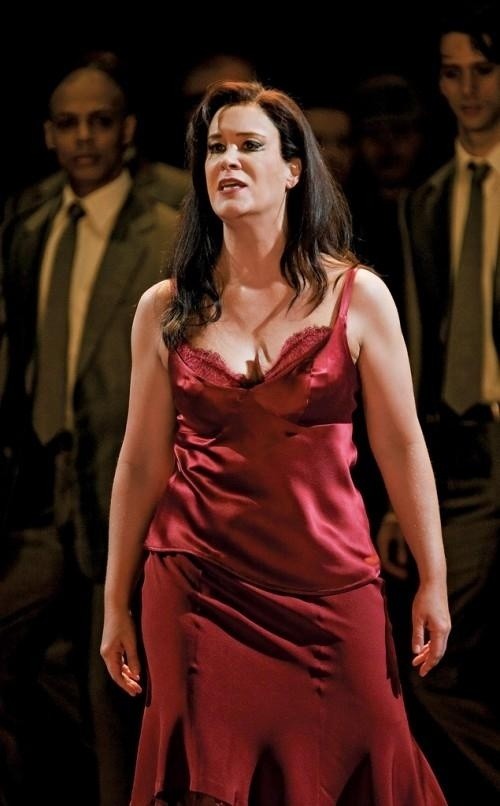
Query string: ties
[34,204,89,446]
[444,161,492,414]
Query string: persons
[1,48,447,580]
[0,65,186,803]
[100,81,452,805]
[373,20,498,804]
